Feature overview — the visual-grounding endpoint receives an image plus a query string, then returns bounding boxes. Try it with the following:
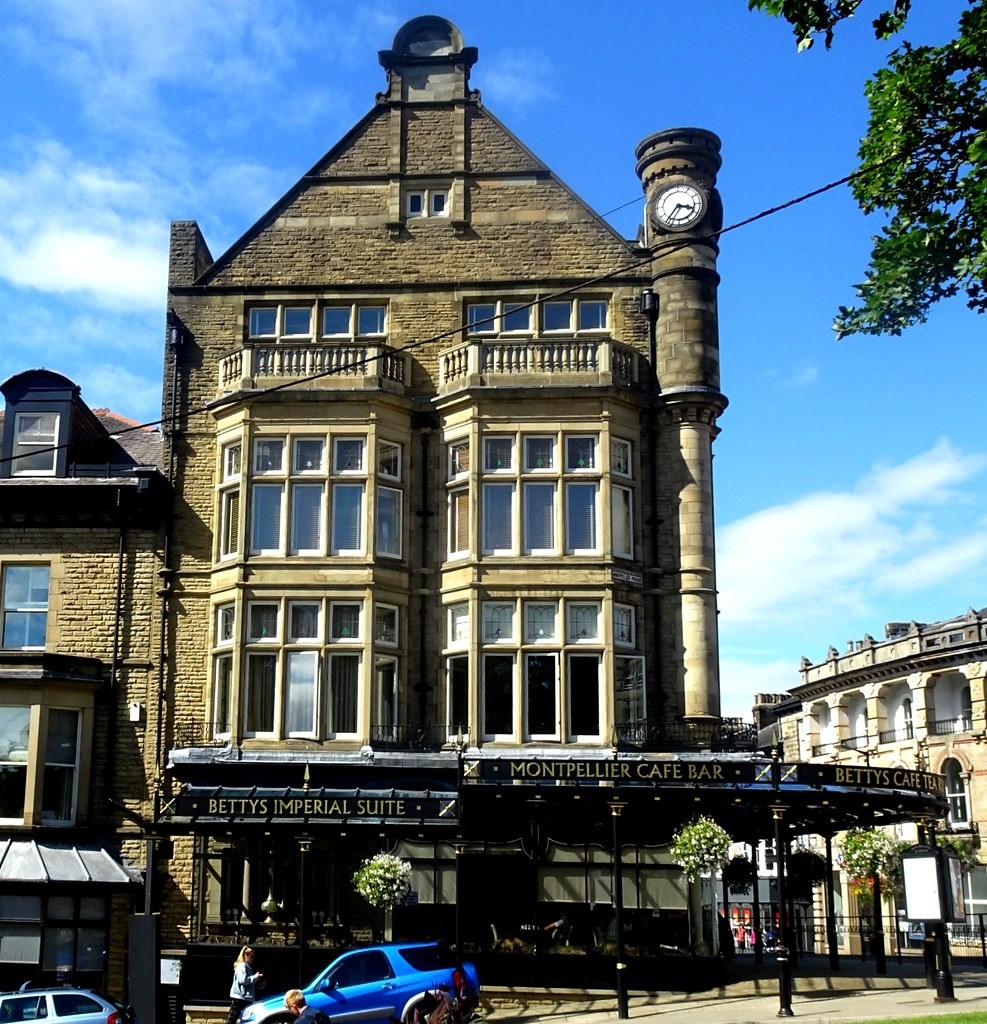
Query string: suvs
[236,941,481,1024]
[0,984,135,1024]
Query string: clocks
[648,181,709,233]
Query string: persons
[283,989,333,1024]
[226,945,263,1024]
[732,921,755,953]
